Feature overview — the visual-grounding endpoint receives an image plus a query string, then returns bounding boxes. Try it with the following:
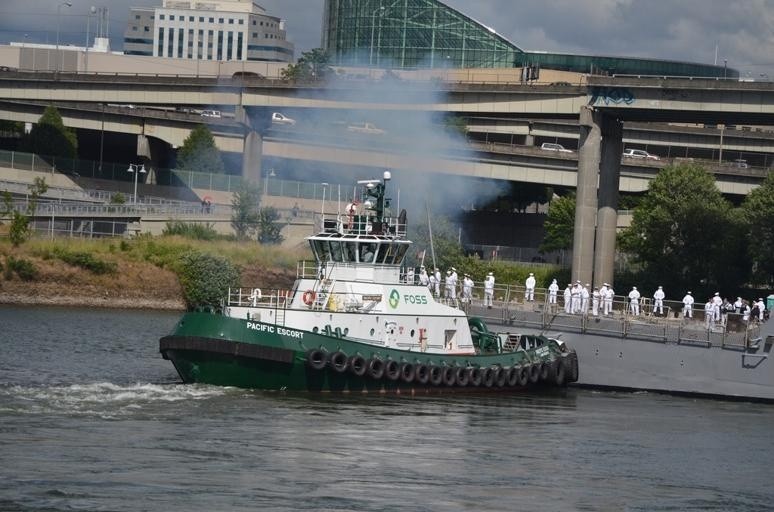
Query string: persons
[564,280,615,316]
[525,273,535,301]
[653,286,665,315]
[406,265,495,306]
[705,292,765,331]
[629,287,640,315]
[201,198,210,213]
[549,279,558,303]
[683,291,694,317]
[364,248,373,262]
[292,203,298,216]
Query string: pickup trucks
[346,120,386,138]
[270,112,297,127]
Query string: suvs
[623,147,658,160]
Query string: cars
[539,141,575,153]
[735,157,750,170]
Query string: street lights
[123,159,148,205]
[83,4,96,76]
[55,2,71,74]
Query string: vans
[198,110,221,118]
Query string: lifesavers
[303,290,315,305]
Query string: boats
[157,171,579,393]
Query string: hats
[529,272,534,276]
[409,265,493,278]
[707,285,763,307]
[687,290,692,295]
[551,273,611,290]
[632,286,638,290]
[658,285,664,290]
